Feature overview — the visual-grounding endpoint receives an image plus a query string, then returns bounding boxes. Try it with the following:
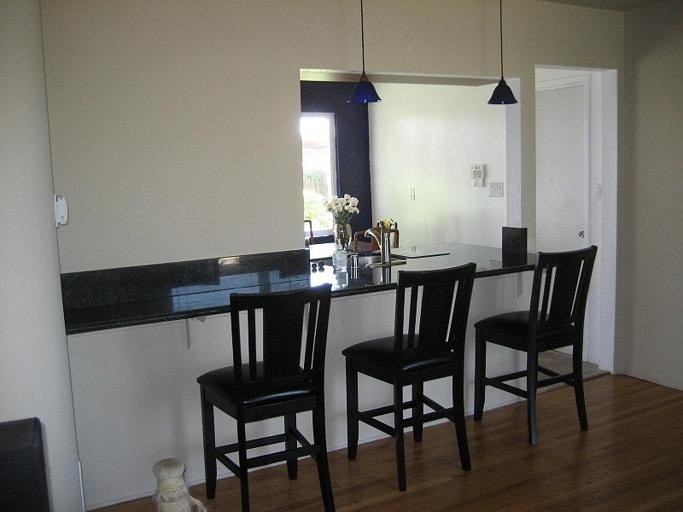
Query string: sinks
[310,255,407,271]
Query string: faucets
[364,266,392,287]
[364,221,390,263]
[304,220,313,237]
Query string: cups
[334,272,347,288]
[332,252,337,266]
[336,251,347,269]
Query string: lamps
[486,0,518,105]
[344,1,382,105]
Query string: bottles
[351,255,358,268]
[350,269,359,280]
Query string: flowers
[323,194,360,247]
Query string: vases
[333,219,352,249]
